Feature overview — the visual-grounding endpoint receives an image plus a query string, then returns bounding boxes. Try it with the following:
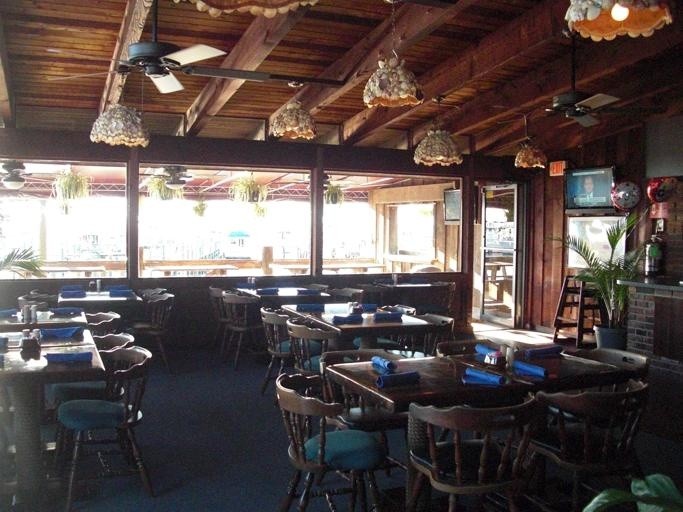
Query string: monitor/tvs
[563,164,616,218]
[442,188,464,226]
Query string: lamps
[89,59,151,148]
[164,180,187,191]
[515,112,548,170]
[1,176,26,191]
[362,0,424,108]
[412,95,464,166]
[269,80,320,140]
[564,0,673,43]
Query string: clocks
[611,180,643,211]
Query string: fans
[139,166,193,180]
[490,26,669,129]
[46,0,271,94]
[0,159,63,180]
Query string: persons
[576,176,603,207]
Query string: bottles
[22,304,38,323]
[89,279,101,291]
[246,276,256,289]
[644,234,665,276]
[18,328,41,347]
[391,272,397,285]
[500,343,514,369]
[346,301,359,313]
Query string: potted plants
[49,165,94,215]
[325,185,345,205]
[139,173,186,207]
[547,210,653,350]
[228,171,271,206]
